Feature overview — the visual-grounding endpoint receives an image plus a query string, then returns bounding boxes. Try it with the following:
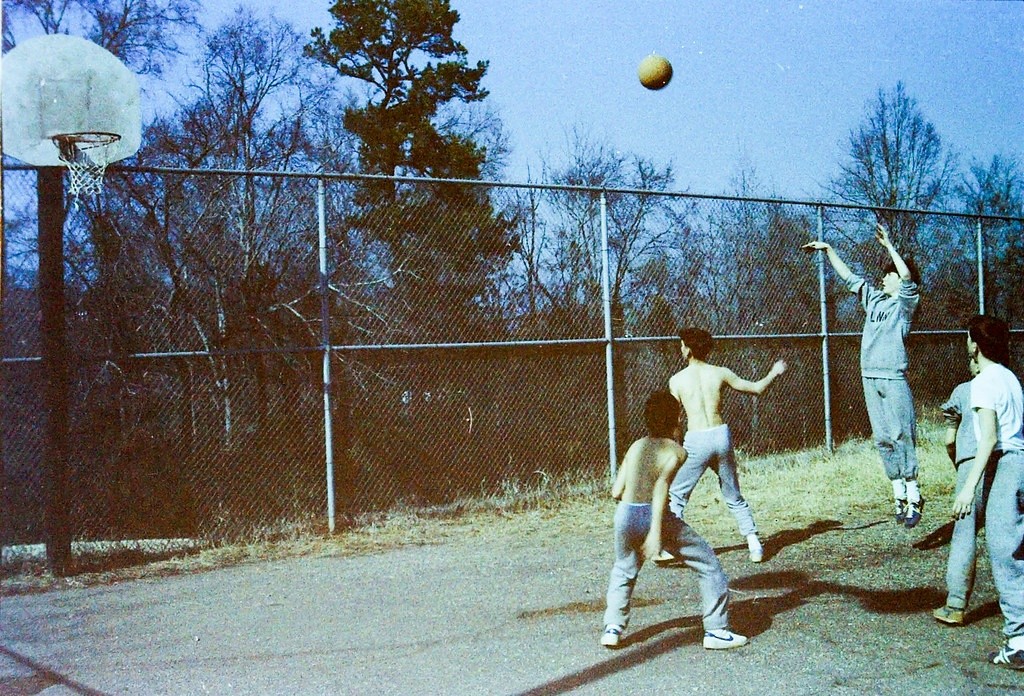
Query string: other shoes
[750,549,763,562]
[652,551,683,566]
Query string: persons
[933,355,984,624]
[651,328,788,562]
[952,315,1024,669]
[802,223,925,529]
[600,390,748,649]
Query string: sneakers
[894,499,907,524]
[989,645,1024,669]
[933,606,964,624]
[903,495,925,528]
[600,629,620,645]
[702,631,748,649]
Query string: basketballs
[636,54,674,91]
